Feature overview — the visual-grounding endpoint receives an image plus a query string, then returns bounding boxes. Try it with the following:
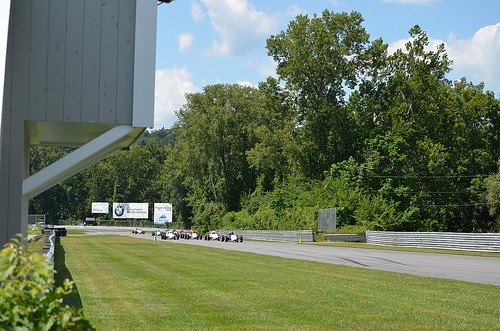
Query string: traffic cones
[298,236,303,244]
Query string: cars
[132,227,244,243]
